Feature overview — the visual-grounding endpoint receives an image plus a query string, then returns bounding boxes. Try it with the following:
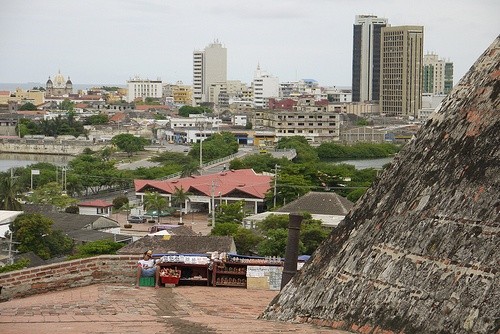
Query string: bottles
[216,256,283,287]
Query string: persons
[134,250,161,289]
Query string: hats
[144,249,154,258]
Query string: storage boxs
[160,269,181,285]
[138,276,155,287]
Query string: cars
[128,216,147,224]
